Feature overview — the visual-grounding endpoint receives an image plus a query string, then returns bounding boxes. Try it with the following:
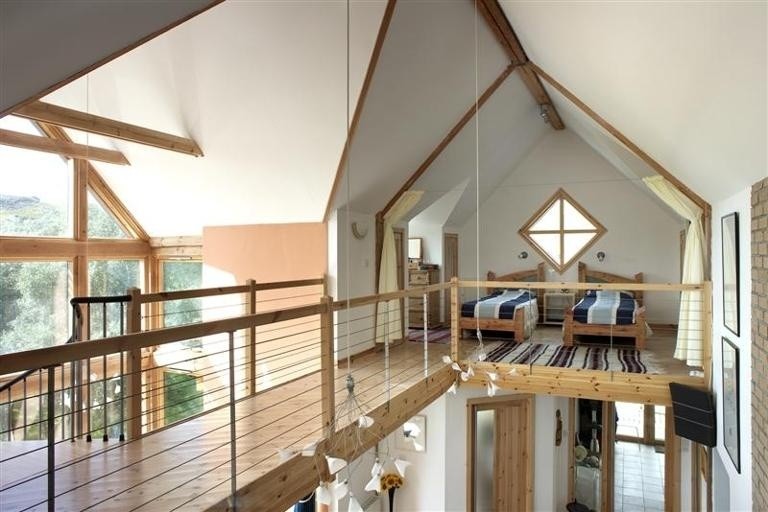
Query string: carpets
[409,326,451,343]
[465,339,663,376]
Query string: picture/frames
[720,209,738,471]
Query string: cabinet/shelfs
[406,270,441,330]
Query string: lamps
[441,0,523,399]
[292,2,422,512]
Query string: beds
[460,261,545,343]
[563,261,647,350]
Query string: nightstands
[543,291,575,326]
